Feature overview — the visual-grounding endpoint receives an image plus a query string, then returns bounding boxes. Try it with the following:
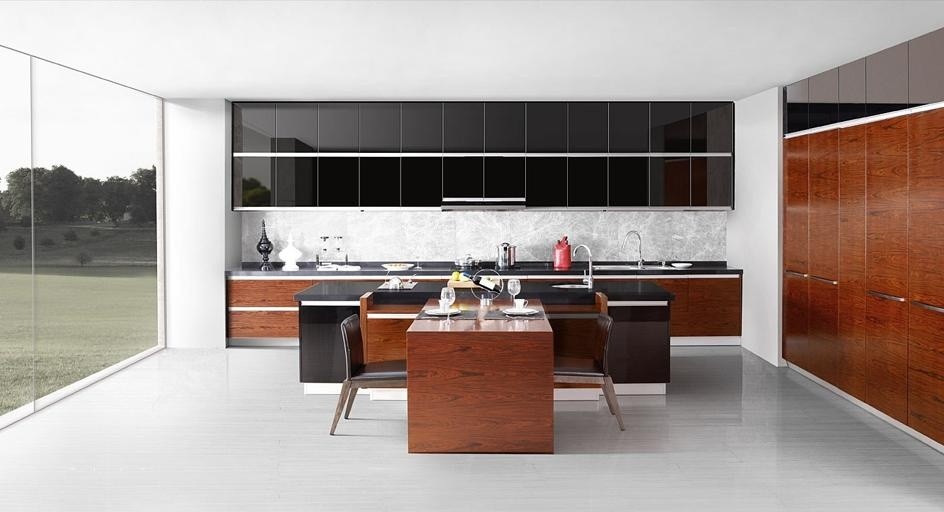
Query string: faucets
[573,244,595,290]
[621,230,645,267]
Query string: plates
[671,262,692,269]
[502,308,539,316]
[381,263,413,270]
[424,308,462,316]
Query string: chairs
[554,313,627,431]
[329,314,408,435]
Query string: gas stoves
[451,261,520,271]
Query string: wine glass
[506,280,521,310]
[440,287,455,325]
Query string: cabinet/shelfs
[605,301,670,395]
[298,301,360,395]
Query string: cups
[439,300,449,311]
[513,299,528,309]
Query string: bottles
[461,271,502,296]
[315,235,347,266]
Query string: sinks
[593,265,679,270]
[551,282,589,289]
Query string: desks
[406,298,554,452]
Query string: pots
[454,254,479,267]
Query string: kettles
[497,242,516,269]
